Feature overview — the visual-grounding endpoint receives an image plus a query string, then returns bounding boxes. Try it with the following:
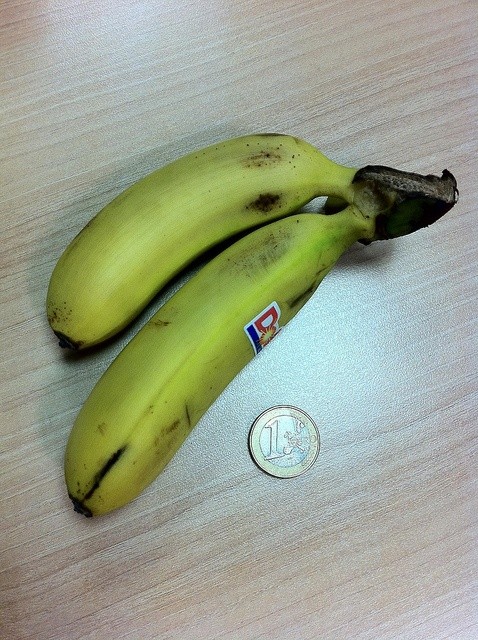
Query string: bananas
[47,131,460,524]
